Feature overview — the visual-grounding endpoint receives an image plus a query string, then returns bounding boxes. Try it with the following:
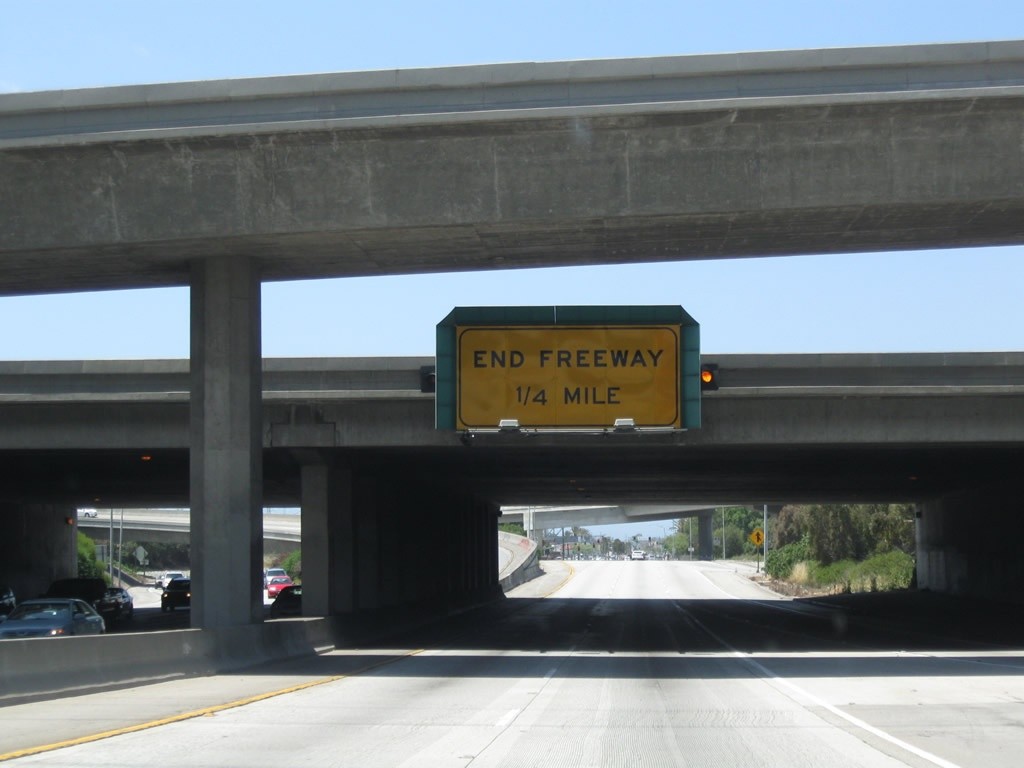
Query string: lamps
[499,419,520,432]
[613,418,636,430]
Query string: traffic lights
[420,365,436,393]
[700,365,718,392]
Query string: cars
[1,576,133,643]
[77,509,97,518]
[263,568,302,618]
[631,550,644,560]
[155,571,190,613]
